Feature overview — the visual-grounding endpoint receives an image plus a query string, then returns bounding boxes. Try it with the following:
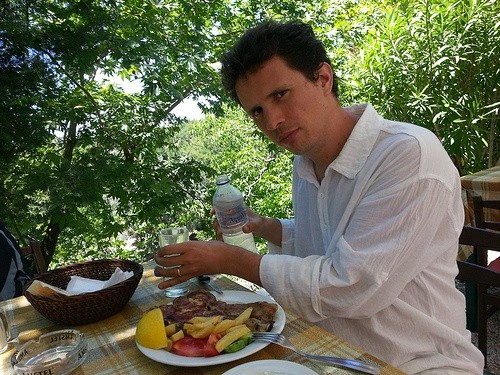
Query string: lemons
[135,308,168,349]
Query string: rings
[177,269,180,276]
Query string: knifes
[199,275,224,294]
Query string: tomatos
[203,333,224,356]
[169,334,208,357]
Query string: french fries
[164,307,253,353]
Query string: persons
[154,19,484,375]
[0,225,28,301]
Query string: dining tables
[458,164,500,262]
[0,267,408,375]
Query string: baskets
[24,259,144,325]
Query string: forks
[251,332,381,375]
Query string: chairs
[454,195,500,375]
[0,239,48,302]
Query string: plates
[135,290,286,367]
[221,360,318,375]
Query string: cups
[0,310,10,354]
[159,228,189,297]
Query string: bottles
[213,175,257,253]
